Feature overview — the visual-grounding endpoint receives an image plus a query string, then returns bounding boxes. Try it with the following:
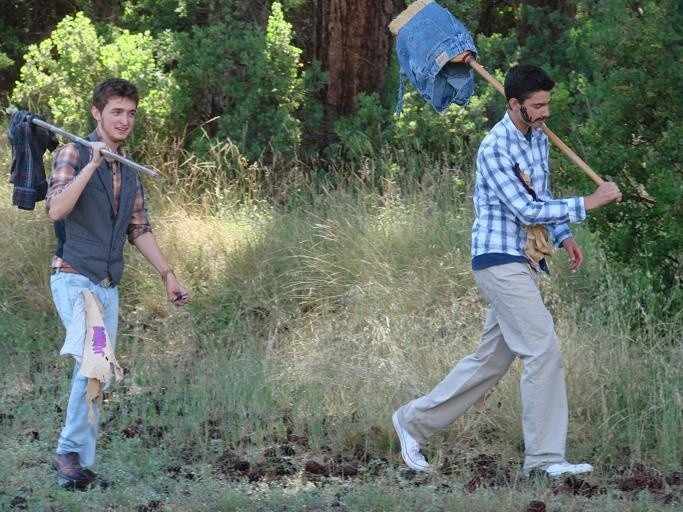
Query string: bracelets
[162,269,174,280]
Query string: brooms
[390,0,622,205]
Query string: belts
[52,266,80,274]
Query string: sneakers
[60,466,114,492]
[389,409,431,471]
[46,451,90,483]
[519,458,595,478]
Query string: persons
[45,80,190,489]
[392,66,623,477]
